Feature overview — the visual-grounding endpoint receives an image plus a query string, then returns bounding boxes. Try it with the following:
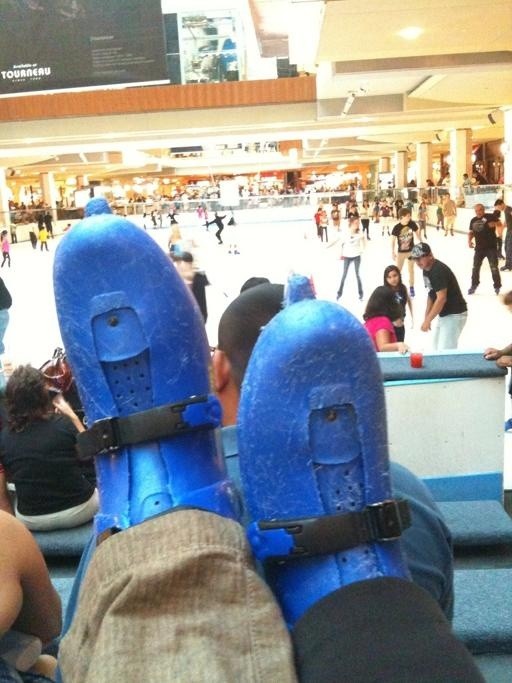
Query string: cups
[410,353,423,369]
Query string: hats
[409,242,431,261]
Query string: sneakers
[318,228,342,243]
[450,231,454,236]
[444,232,448,237]
[409,288,416,297]
[500,265,512,271]
[497,255,506,260]
[235,298,419,656]
[436,226,440,231]
[468,283,479,294]
[366,237,371,240]
[382,232,384,236]
[388,232,390,236]
[336,291,343,299]
[51,213,245,604]
[442,227,445,230]
[359,292,363,299]
[494,283,500,293]
[424,235,428,239]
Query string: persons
[1,172,512,682]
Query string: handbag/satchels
[36,346,75,393]
